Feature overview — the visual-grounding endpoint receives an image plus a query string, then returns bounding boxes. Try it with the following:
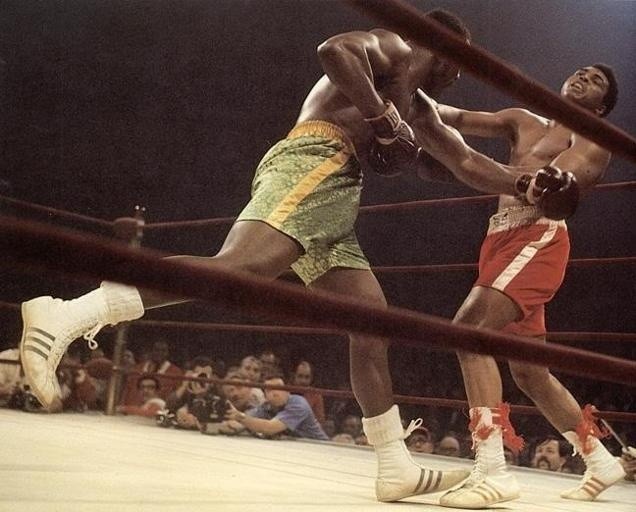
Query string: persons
[406,388,476,460]
[18,7,582,503]
[503,395,636,482]
[417,60,628,511]
[1,337,370,446]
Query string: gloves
[362,98,420,178]
[513,165,579,220]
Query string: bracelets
[237,413,244,422]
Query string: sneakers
[560,460,628,502]
[375,466,471,504]
[19,295,66,412]
[439,472,522,510]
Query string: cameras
[189,372,210,389]
